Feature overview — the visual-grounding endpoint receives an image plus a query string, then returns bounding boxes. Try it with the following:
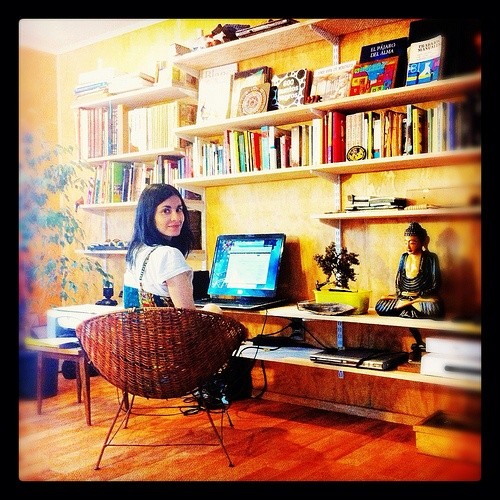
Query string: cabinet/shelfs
[72,16,482,425]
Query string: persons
[122,181,230,409]
[376,220,445,320]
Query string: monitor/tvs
[205,233,284,298]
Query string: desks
[29,337,128,425]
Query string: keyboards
[193,297,268,310]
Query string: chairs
[76,307,245,469]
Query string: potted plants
[313,242,371,315]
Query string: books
[197,19,458,177]
[79,98,196,206]
[73,40,198,102]
[346,193,440,211]
